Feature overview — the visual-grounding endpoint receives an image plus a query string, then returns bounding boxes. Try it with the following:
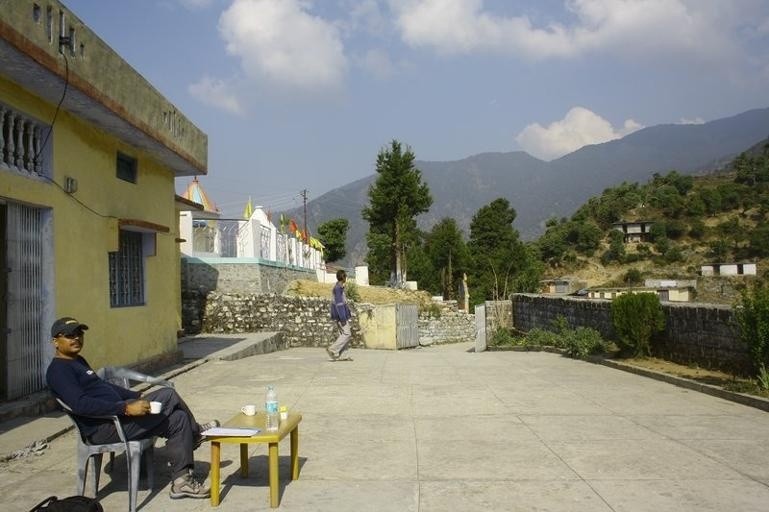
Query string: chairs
[55,368,175,512]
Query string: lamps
[66,176,79,194]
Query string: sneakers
[192,420,220,451]
[169,473,211,499]
[326,348,353,361]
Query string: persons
[45,317,220,499]
[325,269,353,360]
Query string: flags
[243,199,252,219]
[267,210,325,258]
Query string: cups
[241,405,256,416]
[149,402,162,414]
[280,412,288,421]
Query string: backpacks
[29,495,104,512]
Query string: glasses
[64,331,84,339]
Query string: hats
[51,317,88,337]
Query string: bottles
[265,385,279,432]
